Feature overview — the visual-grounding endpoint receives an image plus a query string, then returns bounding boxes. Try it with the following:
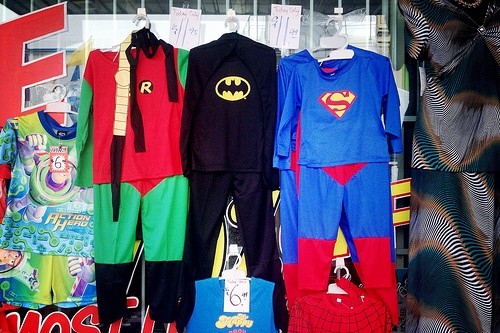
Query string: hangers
[89,12,188,67]
[193,252,275,295]
[7,83,81,143]
[277,17,392,76]
[188,13,277,61]
[291,265,389,319]
